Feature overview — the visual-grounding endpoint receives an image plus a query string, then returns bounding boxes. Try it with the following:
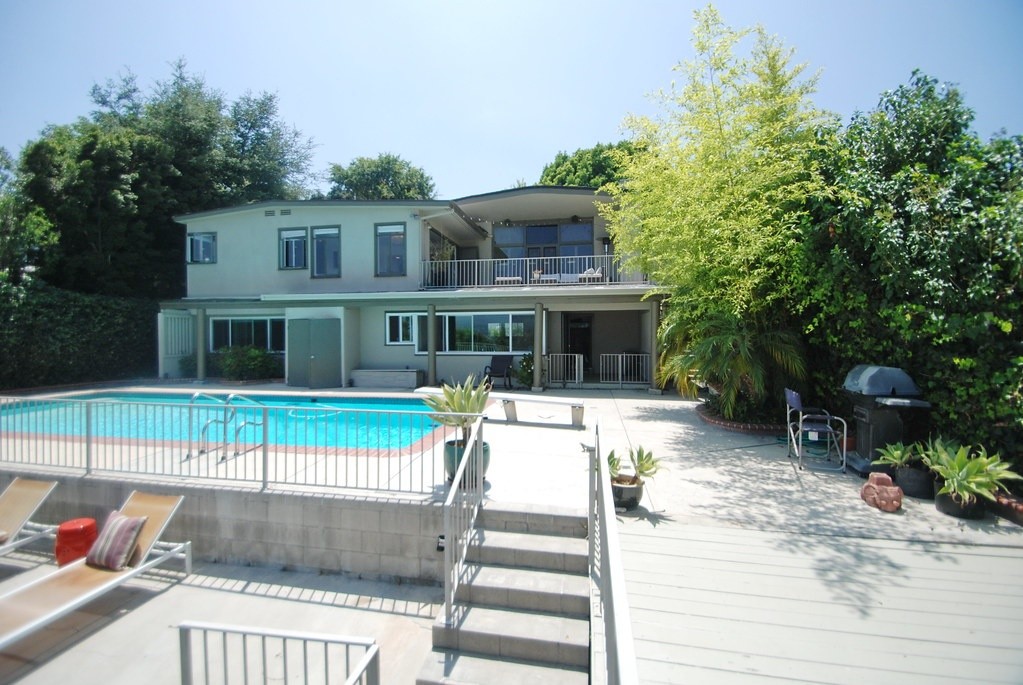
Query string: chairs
[483,354,515,389]
[785,388,847,475]
[0,489,192,652]
[0,477,61,557]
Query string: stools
[57,518,96,568]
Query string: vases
[534,273,540,279]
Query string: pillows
[85,509,149,568]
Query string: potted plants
[516,352,545,389]
[348,379,355,387]
[607,446,658,512]
[420,371,494,487]
[839,428,856,451]
[871,431,1023,520]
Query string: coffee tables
[530,278,558,284]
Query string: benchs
[497,277,522,285]
[540,274,580,282]
[351,369,423,387]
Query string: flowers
[533,269,543,274]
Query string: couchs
[579,267,603,282]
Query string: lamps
[596,227,614,284]
